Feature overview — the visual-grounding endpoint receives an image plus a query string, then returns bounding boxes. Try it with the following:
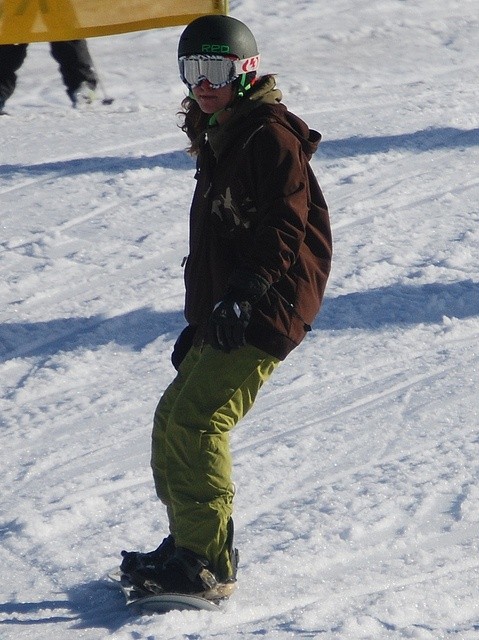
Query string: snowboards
[106,566,223,612]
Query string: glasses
[178,54,258,89]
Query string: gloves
[171,325,197,370]
[207,270,271,354]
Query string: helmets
[178,15,260,96]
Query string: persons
[122,15,334,599]
[1,1,114,115]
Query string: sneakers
[120,535,175,584]
[130,546,240,599]
